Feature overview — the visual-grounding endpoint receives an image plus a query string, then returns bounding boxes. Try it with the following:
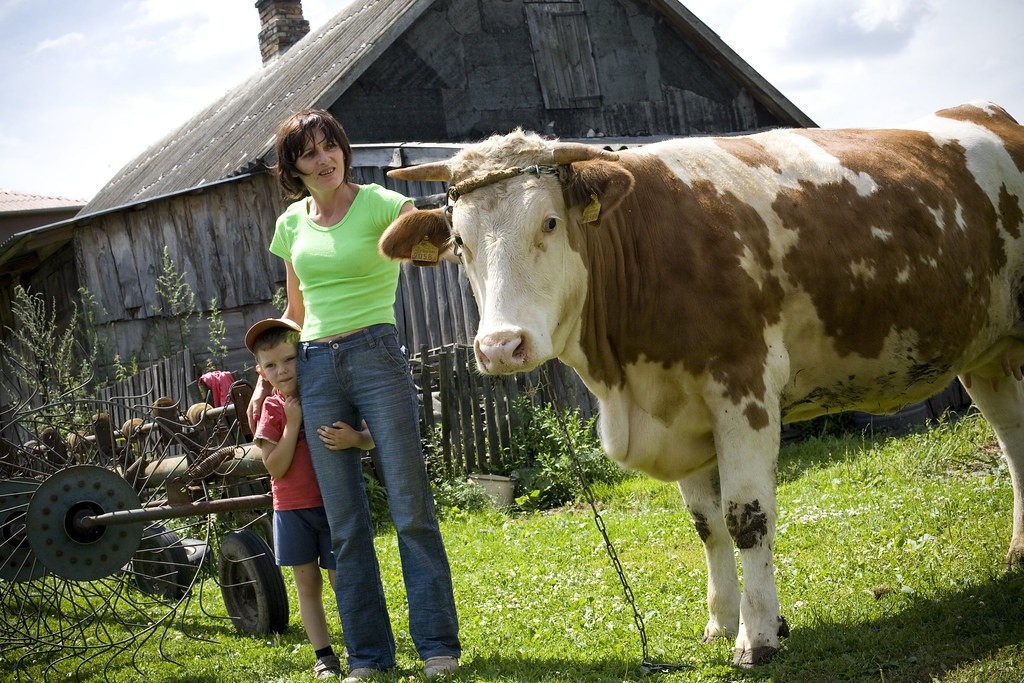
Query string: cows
[378,99,1024,672]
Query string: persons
[243,102,467,683]
[243,316,379,681]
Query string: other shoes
[423,656,458,675]
[313,655,340,680]
[340,668,385,682]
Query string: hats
[245,317,302,354]
